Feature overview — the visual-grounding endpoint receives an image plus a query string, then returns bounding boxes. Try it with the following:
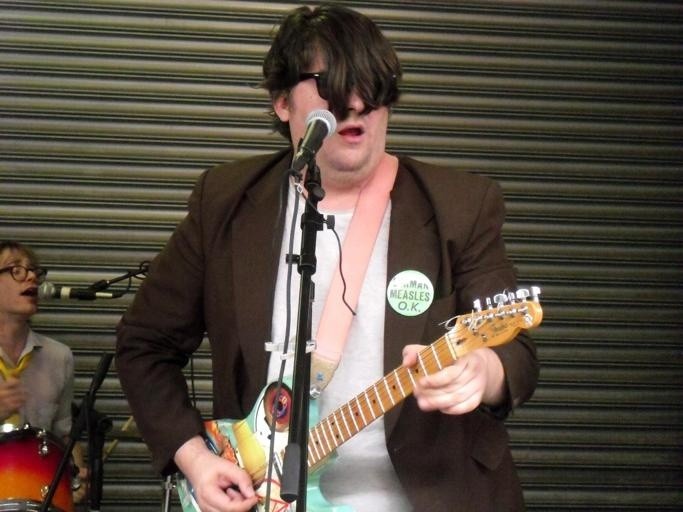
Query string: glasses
[288,71,398,104]
[0,265,47,285]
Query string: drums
[0,422,74,512]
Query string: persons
[0,238,93,511]
[115,2,542,512]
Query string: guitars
[176,285,543,512]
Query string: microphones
[37,281,123,303]
[290,109,338,176]
